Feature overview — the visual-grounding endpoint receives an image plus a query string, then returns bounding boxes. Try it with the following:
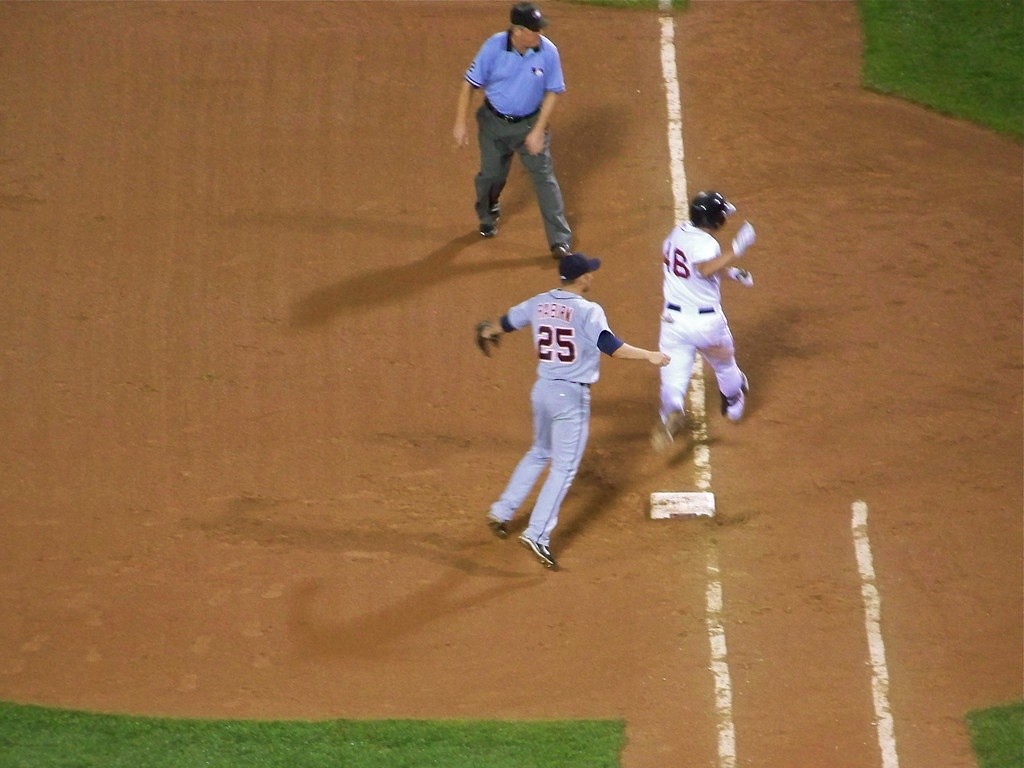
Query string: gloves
[727,266,755,289]
[730,221,756,257]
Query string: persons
[480,253,670,569]
[651,191,757,448]
[454,2,573,259]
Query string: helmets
[689,191,737,227]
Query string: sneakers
[552,246,573,259]
[486,511,508,539]
[517,534,556,569]
[480,223,497,237]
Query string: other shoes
[720,372,748,421]
[650,410,684,452]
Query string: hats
[511,3,549,31]
[559,255,601,281]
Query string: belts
[668,304,715,313]
[485,96,540,123]
[556,378,590,386]
